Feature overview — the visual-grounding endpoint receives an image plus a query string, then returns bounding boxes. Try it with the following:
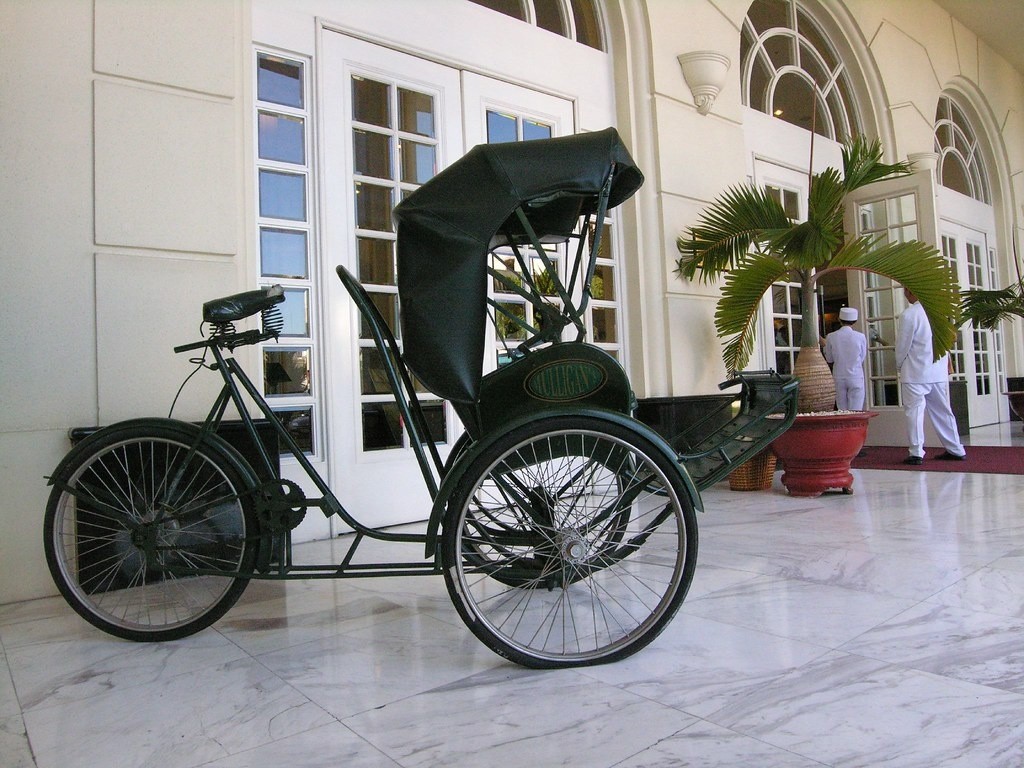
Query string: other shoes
[858,450,867,457]
[903,455,923,465]
[935,449,966,460]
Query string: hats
[839,308,858,321]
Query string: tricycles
[39,126,802,669]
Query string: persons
[869,328,895,352]
[777,325,799,373]
[820,308,867,411]
[896,285,967,465]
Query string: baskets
[728,454,777,491]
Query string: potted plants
[670,127,965,497]
